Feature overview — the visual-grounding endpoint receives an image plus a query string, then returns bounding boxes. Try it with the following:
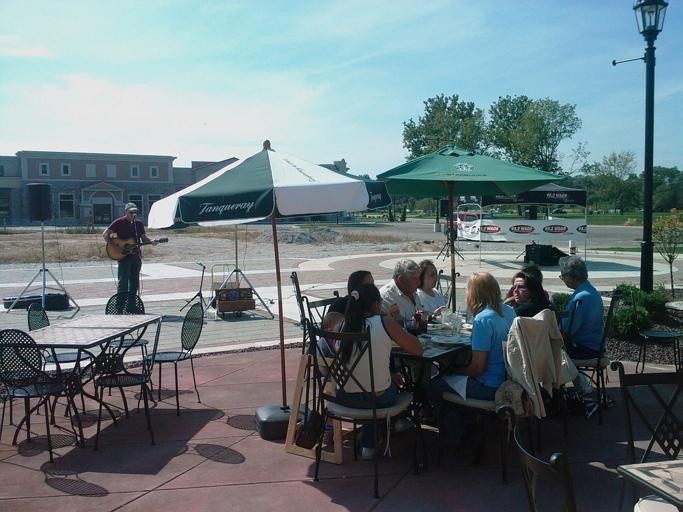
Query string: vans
[445,212,495,243]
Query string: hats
[124,203,139,212]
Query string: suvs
[456,204,495,217]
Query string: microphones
[132,212,137,220]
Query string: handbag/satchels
[296,410,324,450]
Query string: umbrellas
[147,139,392,411]
[376,143,567,313]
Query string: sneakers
[396,419,412,432]
[362,447,374,459]
[584,403,600,418]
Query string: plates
[426,323,444,331]
[432,334,470,345]
[445,323,473,329]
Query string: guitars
[107,237,168,260]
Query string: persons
[103,203,159,314]
[559,256,605,419]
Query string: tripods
[180,263,207,313]
[6,221,80,311]
[203,226,274,318]
[436,217,464,262]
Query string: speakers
[26,183,52,221]
[440,199,449,217]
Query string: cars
[594,207,621,215]
[553,204,593,215]
[104,213,110,223]
[406,209,410,213]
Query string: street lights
[611,1,670,294]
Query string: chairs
[1,291,206,464]
[282,287,683,512]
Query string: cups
[405,320,418,332]
[416,335,430,348]
[440,308,451,332]
